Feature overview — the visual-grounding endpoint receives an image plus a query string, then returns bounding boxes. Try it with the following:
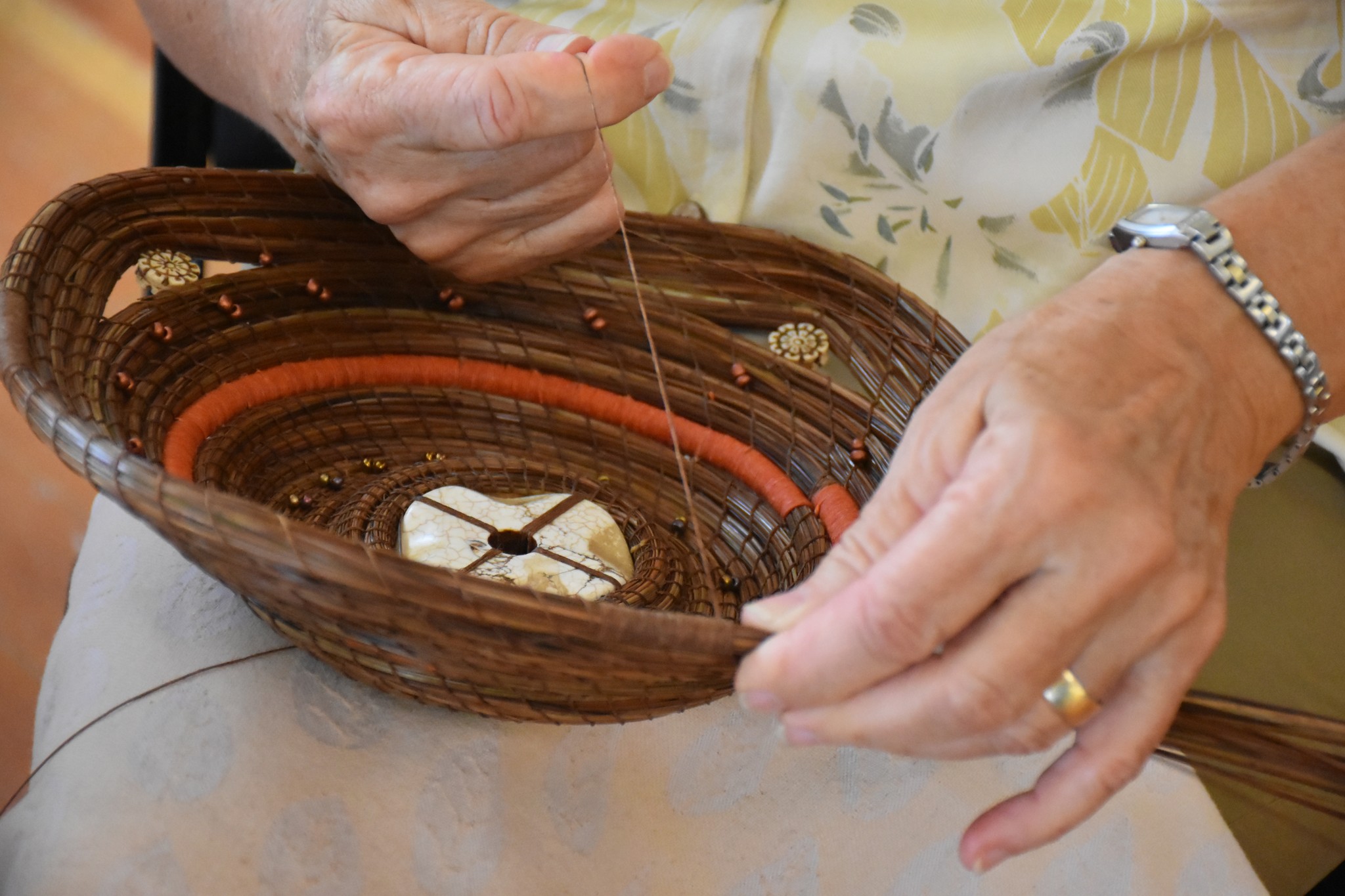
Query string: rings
[1041,665,1101,732]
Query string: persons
[0,0,1344,894]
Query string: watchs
[1109,201,1333,490]
